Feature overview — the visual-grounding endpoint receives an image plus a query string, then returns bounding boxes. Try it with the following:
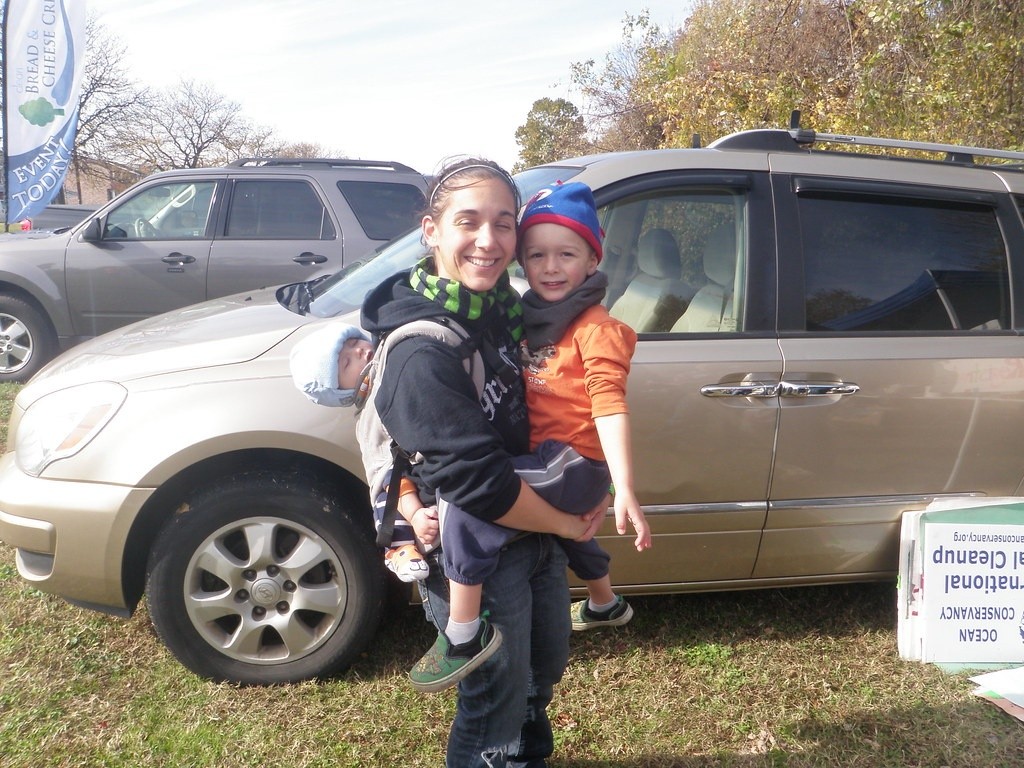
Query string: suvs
[0,111,1024,686]
[0,155,433,383]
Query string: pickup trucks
[20,203,152,231]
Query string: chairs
[607,227,692,333]
[670,225,735,333]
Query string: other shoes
[383,543,430,584]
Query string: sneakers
[408,620,503,693]
[570,597,634,631]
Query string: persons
[289,159,653,768]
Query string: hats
[514,182,605,264]
[290,324,371,408]
